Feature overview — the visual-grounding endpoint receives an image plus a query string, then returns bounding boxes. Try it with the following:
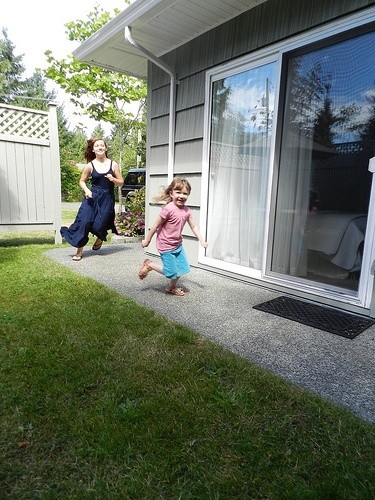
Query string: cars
[121,169,147,203]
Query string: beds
[250,207,368,280]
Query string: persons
[138,177,208,296]
[60,138,123,261]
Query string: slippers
[72,255,82,261]
[92,242,102,250]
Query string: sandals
[138,259,153,280]
[165,284,184,295]
